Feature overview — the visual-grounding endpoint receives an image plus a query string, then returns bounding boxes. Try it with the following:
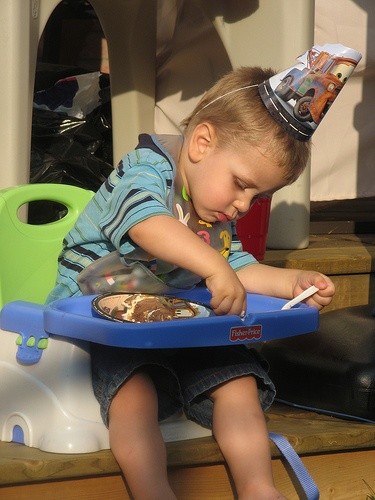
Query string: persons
[46,67,335,500]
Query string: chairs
[0,183,320,454]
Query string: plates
[91,292,211,324]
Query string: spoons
[281,286,319,309]
